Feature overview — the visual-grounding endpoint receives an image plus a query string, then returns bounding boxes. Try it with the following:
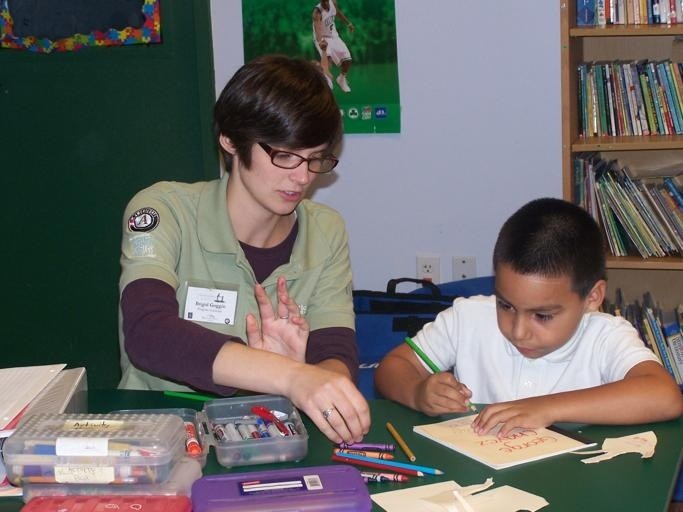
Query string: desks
[0,387,683,512]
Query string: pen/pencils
[405,337,479,414]
[14,436,163,484]
[386,422,416,462]
[331,442,444,481]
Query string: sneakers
[337,76,351,92]
[325,74,333,89]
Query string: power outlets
[416,254,443,284]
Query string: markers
[214,406,299,466]
[184,421,201,458]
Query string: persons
[376,197,683,438]
[313,0,355,93]
[118,56,372,446]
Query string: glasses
[258,140,339,173]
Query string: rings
[279,316,288,319]
[324,408,334,420]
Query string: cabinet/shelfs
[561,1,683,386]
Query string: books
[576,58,683,137]
[413,414,598,471]
[599,288,682,386]
[574,1,681,27]
[576,152,682,257]
